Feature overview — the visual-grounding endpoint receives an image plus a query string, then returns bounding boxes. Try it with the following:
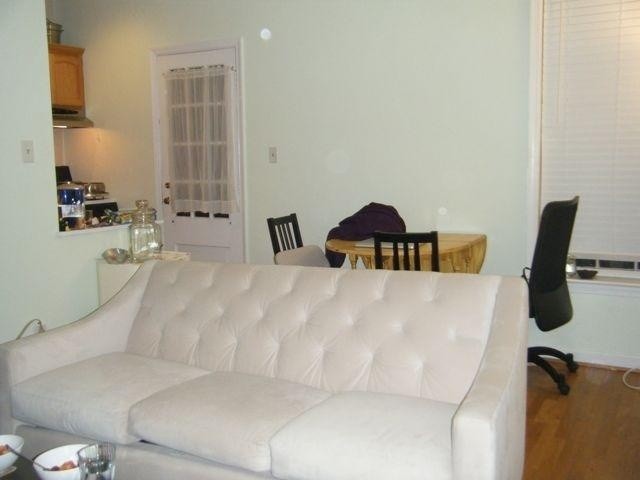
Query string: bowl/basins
[58,189,85,206]
[87,182,109,199]
[1,433,98,479]
[101,248,129,264]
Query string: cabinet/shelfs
[57,218,165,325]
[97,250,189,310]
[48,41,87,109]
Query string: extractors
[52,105,95,129]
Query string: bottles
[131,198,161,262]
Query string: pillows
[275,244,331,268]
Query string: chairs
[372,228,440,272]
[522,194,580,396]
[267,213,304,254]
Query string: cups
[78,444,118,479]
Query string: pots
[48,17,66,46]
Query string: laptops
[351,237,425,250]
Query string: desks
[326,232,487,273]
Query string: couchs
[1,258,530,480]
